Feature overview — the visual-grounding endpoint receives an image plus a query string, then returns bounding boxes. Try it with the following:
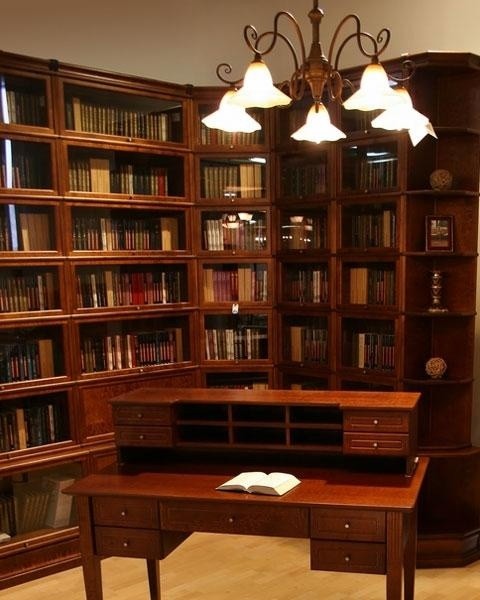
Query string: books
[0,80,71,538]
[63,95,187,375]
[272,88,396,371]
[198,114,269,362]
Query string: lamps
[201,0,439,148]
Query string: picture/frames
[425,215,454,252]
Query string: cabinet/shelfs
[106,386,422,486]
[0,49,199,589]
[190,81,273,390]
[272,52,480,570]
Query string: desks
[62,456,431,600]
